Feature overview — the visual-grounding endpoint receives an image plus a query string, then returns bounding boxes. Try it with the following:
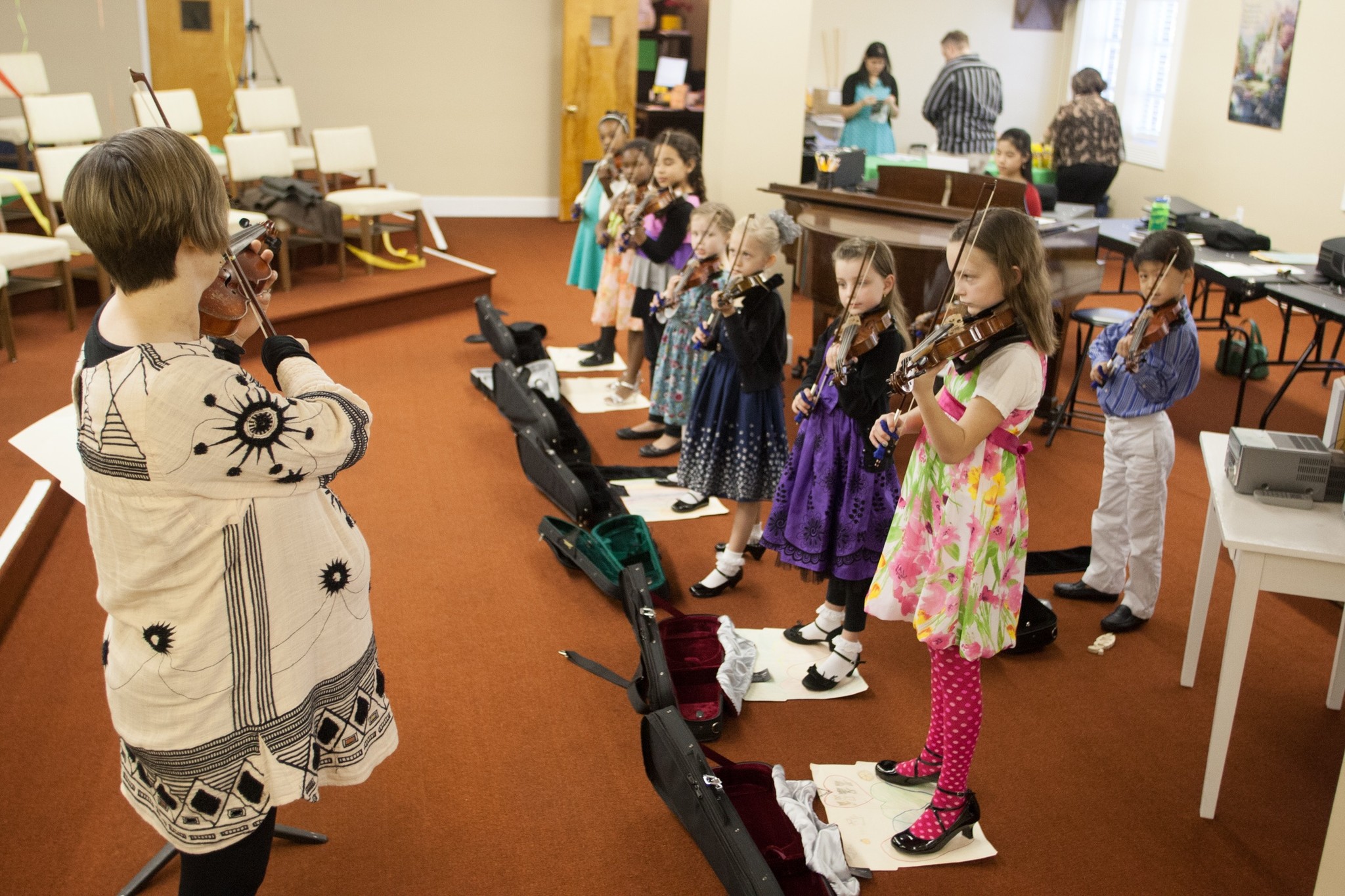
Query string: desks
[1068,218,1330,365]
[1178,431,1345,819]
[1258,282,1345,428]
[635,102,704,142]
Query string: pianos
[755,164,1105,435]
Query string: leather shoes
[1101,605,1148,632]
[616,420,663,438]
[1054,579,1119,602]
[671,493,709,512]
[640,440,681,456]
[656,476,682,487]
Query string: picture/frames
[1228,0,1300,130]
[1012,0,1065,32]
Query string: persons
[60,128,397,896]
[567,30,1197,855]
[1256,23,1298,81]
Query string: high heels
[891,785,980,853]
[603,371,641,405]
[783,617,843,651]
[876,744,944,786]
[802,649,866,691]
[715,542,766,561]
[689,563,743,598]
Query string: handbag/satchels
[1215,319,1269,379]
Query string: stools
[1045,307,1135,447]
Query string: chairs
[0,51,425,364]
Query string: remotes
[1254,490,1313,509]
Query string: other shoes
[579,340,616,351]
[579,352,614,365]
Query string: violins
[1124,300,1184,375]
[884,298,1017,397]
[664,256,725,310]
[716,270,770,309]
[595,151,623,181]
[617,185,685,254]
[197,218,278,339]
[824,306,893,388]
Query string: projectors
[1225,427,1329,500]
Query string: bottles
[1148,194,1170,234]
